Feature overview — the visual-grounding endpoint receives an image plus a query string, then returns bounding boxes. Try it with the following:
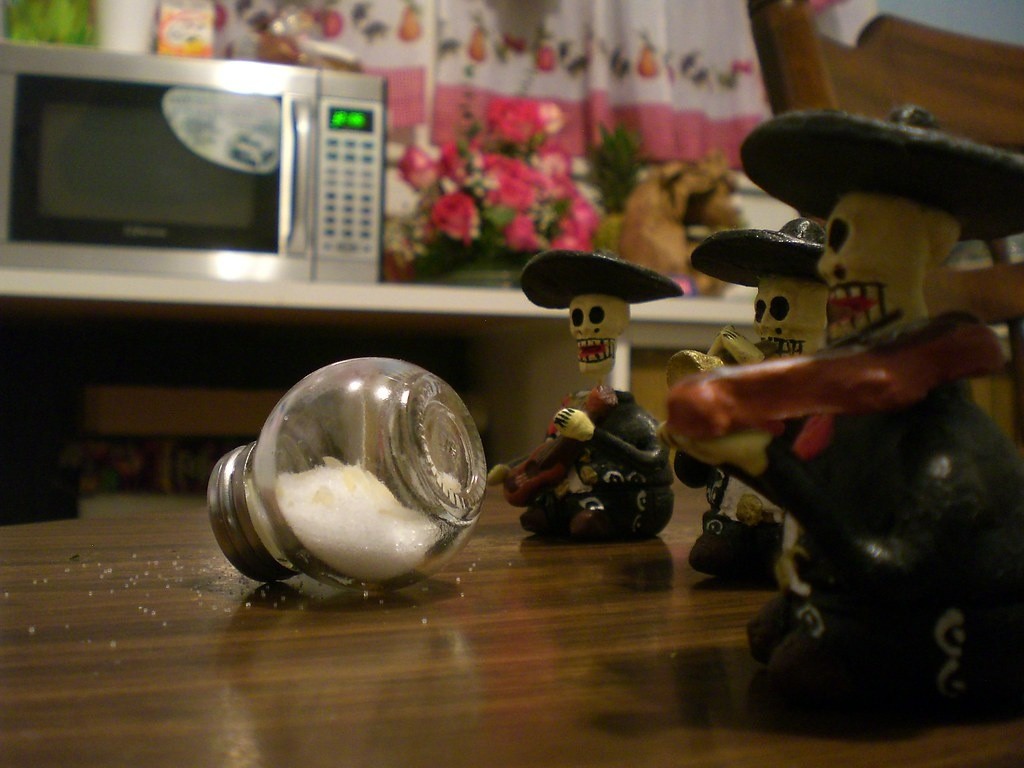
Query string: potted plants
[567,118,652,259]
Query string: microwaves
[0,39,387,283]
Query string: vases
[437,267,525,292]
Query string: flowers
[373,96,604,279]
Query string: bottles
[207,357,486,592]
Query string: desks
[0,467,1023,768]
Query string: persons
[655,218,830,590]
[484,248,684,543]
[742,102,1024,714]
